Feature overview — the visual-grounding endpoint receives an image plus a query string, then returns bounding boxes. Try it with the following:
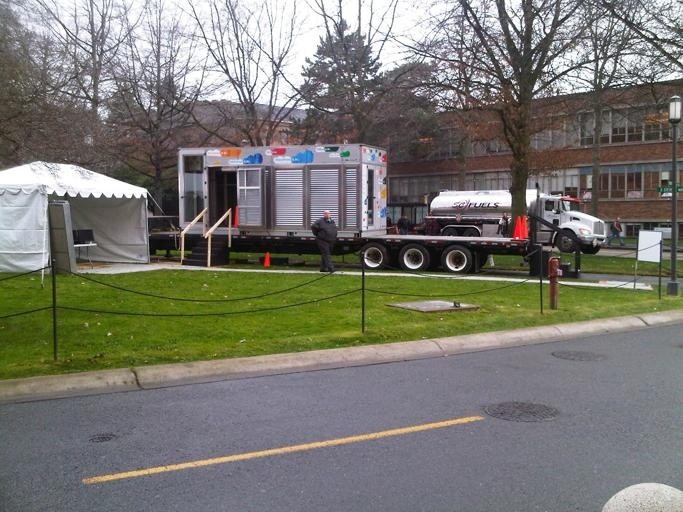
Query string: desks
[74,243,97,271]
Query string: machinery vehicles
[423,188,610,255]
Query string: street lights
[665,93,682,296]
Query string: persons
[311,210,338,273]
[397,215,410,235]
[387,213,391,227]
[607,216,625,246]
[496,210,512,238]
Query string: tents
[0,160,152,276]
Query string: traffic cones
[263,251,271,267]
